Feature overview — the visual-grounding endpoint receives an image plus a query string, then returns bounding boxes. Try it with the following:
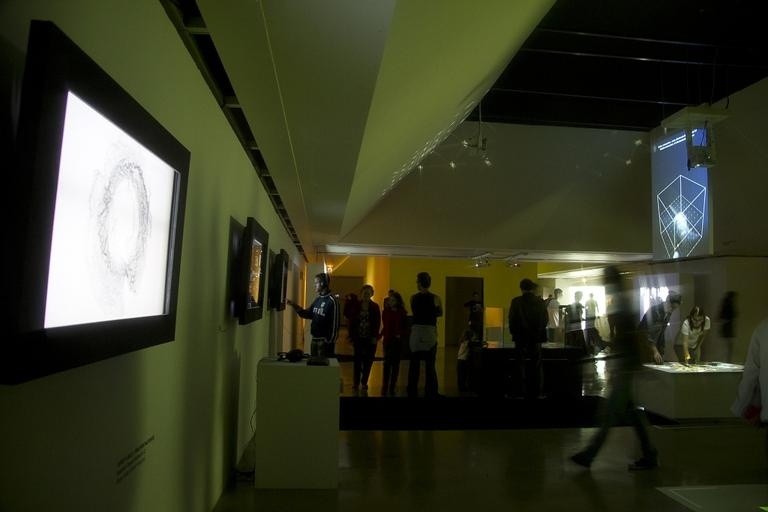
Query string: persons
[464,292,484,341]
[456,329,473,391]
[346,285,380,391]
[382,293,409,395]
[384,290,394,309]
[344,293,358,339]
[717,289,738,364]
[410,272,442,394]
[285,273,340,358]
[729,314,767,433]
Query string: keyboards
[308,359,329,366]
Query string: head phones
[278,349,304,362]
[318,274,327,288]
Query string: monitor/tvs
[0,19,190,389]
[276,249,289,312]
[239,218,269,326]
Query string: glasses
[691,318,705,323]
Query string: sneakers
[627,457,658,471]
[568,453,594,468]
